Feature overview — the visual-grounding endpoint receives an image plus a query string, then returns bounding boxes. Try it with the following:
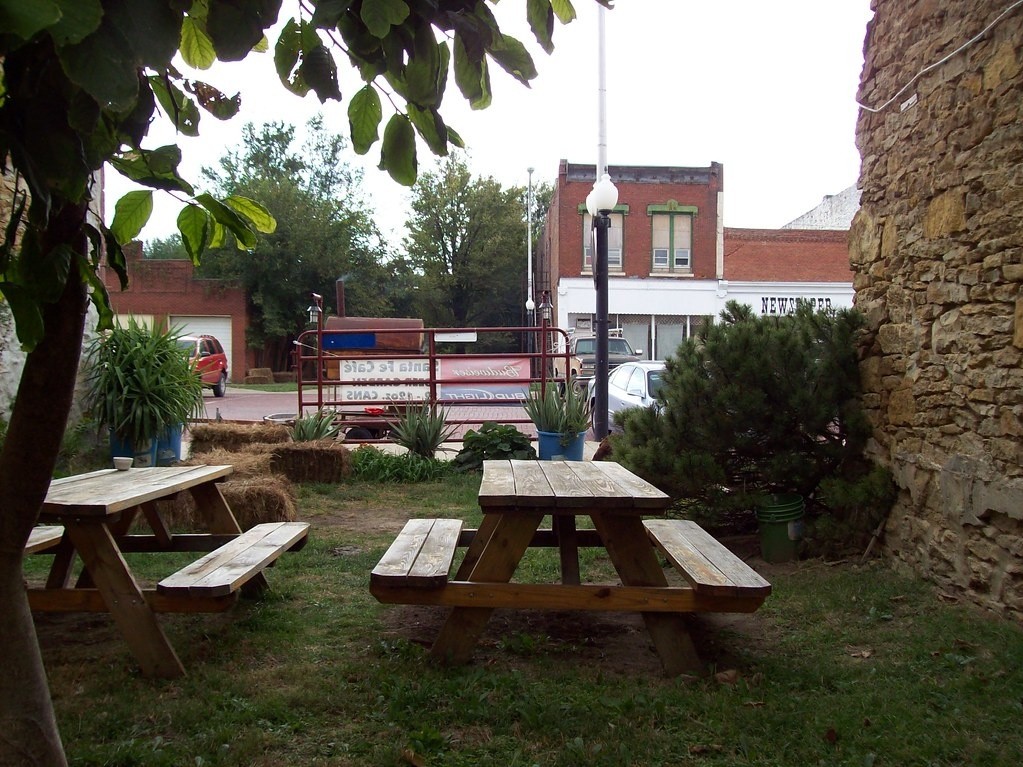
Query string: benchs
[370,518,463,607]
[639,517,772,612]
[156,522,311,599]
[23,525,65,555]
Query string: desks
[431,459,710,681]
[36,464,269,682]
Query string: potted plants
[82,304,222,468]
[518,371,598,461]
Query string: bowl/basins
[114,457,133,470]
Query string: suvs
[551,327,642,403]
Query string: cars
[586,359,680,435]
[168,334,228,398]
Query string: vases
[709,491,804,562]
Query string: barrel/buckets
[758,494,806,564]
